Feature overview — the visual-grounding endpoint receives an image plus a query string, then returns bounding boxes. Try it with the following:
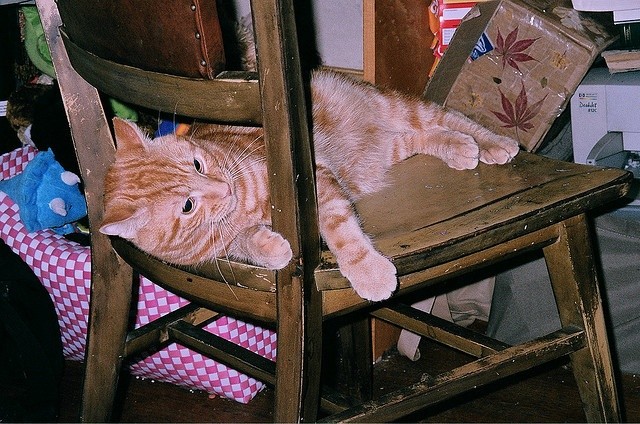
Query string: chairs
[34,1,637,423]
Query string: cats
[95,65,523,306]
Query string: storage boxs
[420,1,623,157]
[569,66,639,205]
